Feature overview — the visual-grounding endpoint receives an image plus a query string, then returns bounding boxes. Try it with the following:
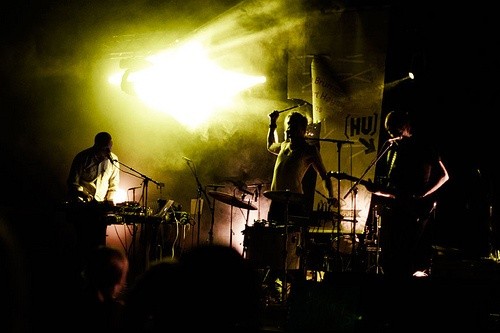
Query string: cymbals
[207,190,258,209]
[228,179,254,195]
[262,190,305,201]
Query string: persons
[267,110,335,225]
[67,131,119,202]
[48,246,486,333]
[366,111,450,281]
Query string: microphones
[388,136,403,142]
[284,130,290,141]
[128,187,142,190]
[207,184,226,188]
[106,153,114,164]
[235,186,254,196]
[182,156,192,163]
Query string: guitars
[326,171,441,232]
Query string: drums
[258,270,304,306]
[280,226,314,271]
[243,223,294,255]
